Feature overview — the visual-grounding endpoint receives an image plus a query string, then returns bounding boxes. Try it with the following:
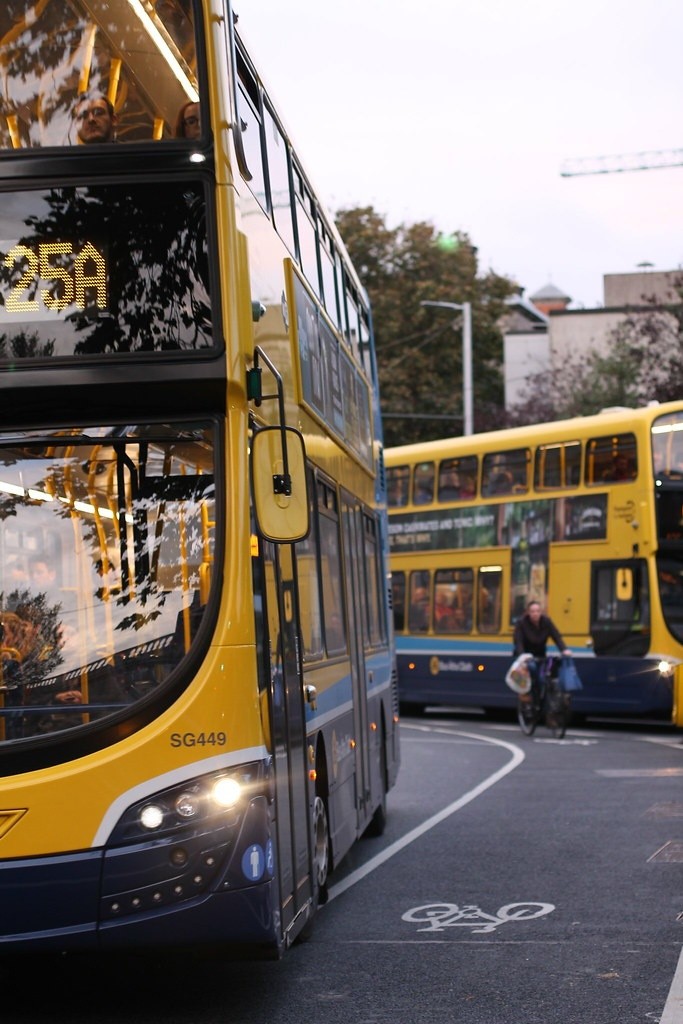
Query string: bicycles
[515,648,574,741]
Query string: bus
[0,0,403,989]
[382,396,683,720]
[264,433,382,686]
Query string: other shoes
[518,694,532,702]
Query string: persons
[75,92,114,145]
[385,436,634,505]
[325,613,344,651]
[512,600,571,727]
[392,584,495,634]
[0,554,80,653]
[175,101,202,140]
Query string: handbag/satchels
[558,655,583,691]
[505,653,534,694]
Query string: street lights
[419,300,476,438]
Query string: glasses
[79,107,105,118]
[183,116,199,127]
